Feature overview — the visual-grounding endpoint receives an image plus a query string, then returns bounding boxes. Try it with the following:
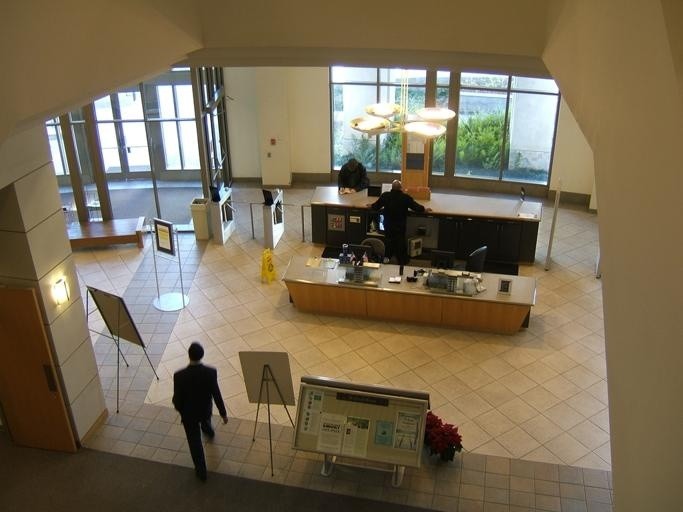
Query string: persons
[365,179,432,266]
[170,340,229,484]
[337,157,369,193]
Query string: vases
[440,446,456,462]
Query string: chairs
[465,246,488,273]
[361,238,385,263]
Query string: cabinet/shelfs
[311,205,539,265]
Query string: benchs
[69,216,146,248]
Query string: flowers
[424,412,463,454]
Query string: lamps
[52,278,70,305]
[349,69,456,137]
